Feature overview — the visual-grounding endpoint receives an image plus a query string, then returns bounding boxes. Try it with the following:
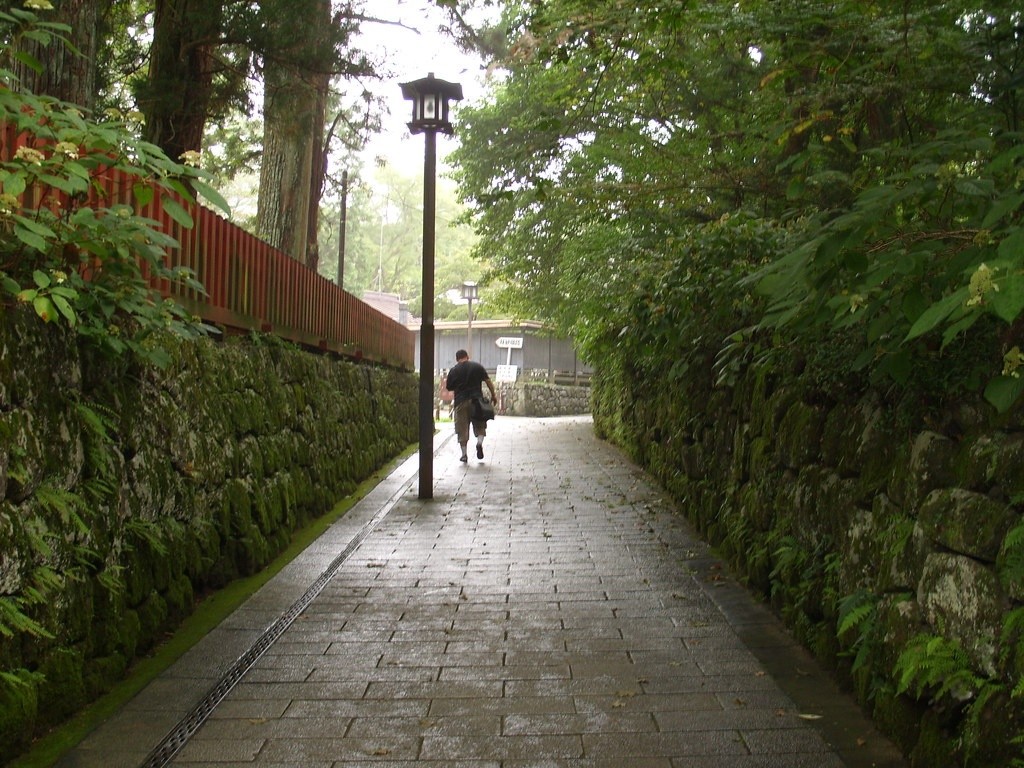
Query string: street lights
[398,73,464,501]
[460,280,479,361]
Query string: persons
[446,349,497,462]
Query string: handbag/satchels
[477,397,494,420]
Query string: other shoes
[460,456,467,462]
[476,444,483,459]
